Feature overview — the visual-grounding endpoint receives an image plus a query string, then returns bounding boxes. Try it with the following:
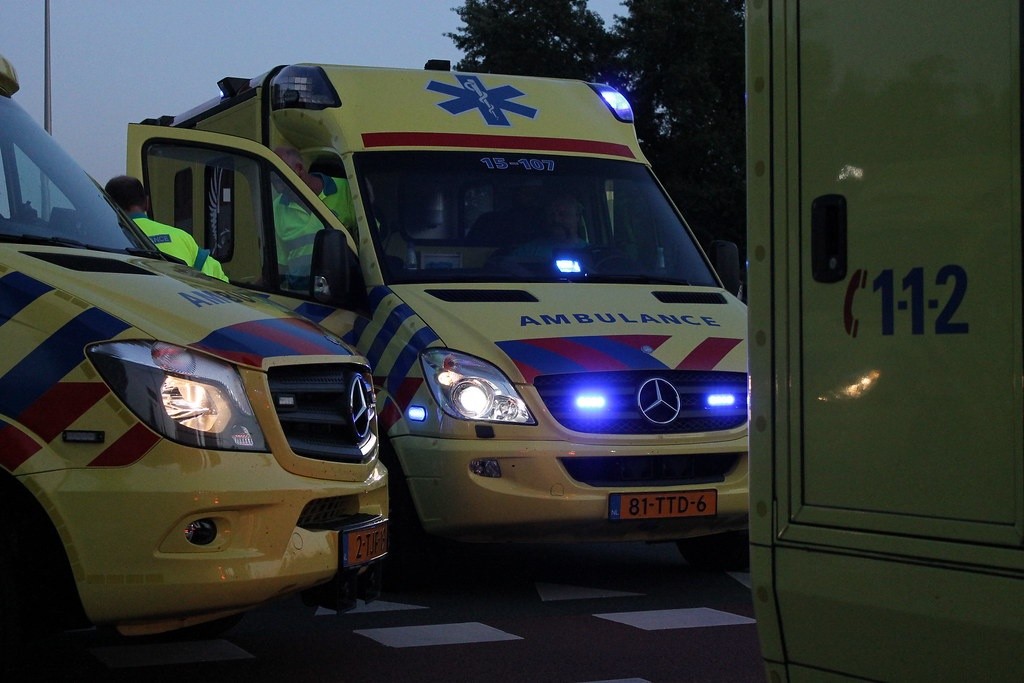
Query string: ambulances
[124,59,751,573]
[0,53,392,658]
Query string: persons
[103,176,230,283]
[269,147,359,302]
[465,165,571,245]
